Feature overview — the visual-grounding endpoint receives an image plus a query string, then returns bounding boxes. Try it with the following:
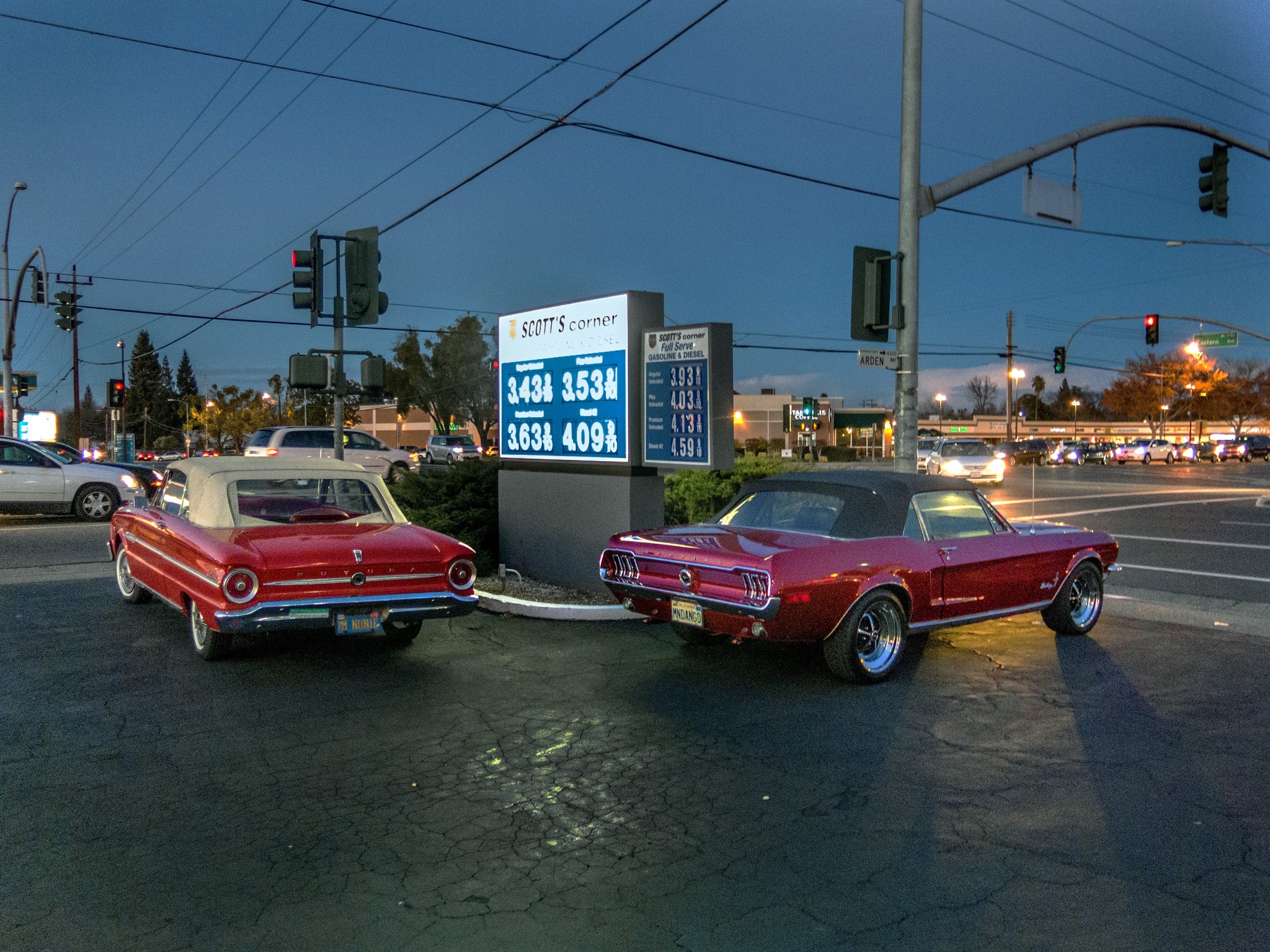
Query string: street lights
[935,394,947,437]
[346,226,389,325]
[292,248,316,312]
[1071,400,1080,441]
[2,182,49,437]
[1161,404,1168,439]
[1185,384,1195,443]
[168,399,189,457]
[1009,369,1025,439]
[116,338,127,463]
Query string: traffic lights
[17,376,28,396]
[109,379,124,407]
[31,269,49,304]
[70,293,83,328]
[814,400,820,416]
[803,397,811,417]
[53,291,71,330]
[1198,142,1229,214]
[1145,315,1158,344]
[1054,347,1064,371]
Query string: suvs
[992,440,1050,467]
[426,436,484,465]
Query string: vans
[244,427,421,484]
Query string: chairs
[238,497,321,513]
[904,509,933,538]
[795,505,838,532]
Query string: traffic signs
[857,349,899,370]
[1193,332,1238,348]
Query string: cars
[918,437,953,473]
[108,456,479,659]
[192,450,219,457]
[0,436,145,522]
[485,446,499,458]
[135,452,153,461]
[27,441,163,499]
[394,446,419,453]
[154,452,187,461]
[599,470,1118,683]
[1041,434,1268,465]
[925,439,1005,485]
[414,448,427,459]
